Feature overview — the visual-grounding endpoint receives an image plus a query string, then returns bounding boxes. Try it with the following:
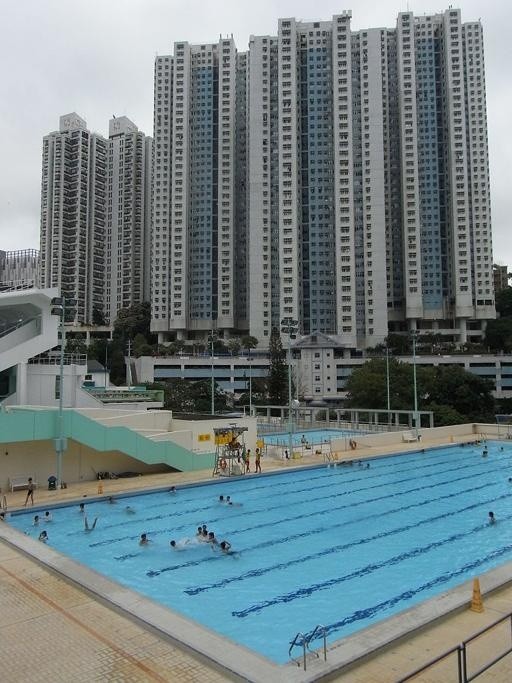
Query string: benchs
[403,430,424,443]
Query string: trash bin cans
[447,435,453,443]
[48,476,57,489]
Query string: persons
[304,441,312,450]
[245,449,251,474]
[229,437,243,450]
[253,448,264,474]
[300,434,307,443]
[487,511,495,524]
[459,439,505,457]
[337,459,371,470]
[31,486,235,561]
[21,477,35,507]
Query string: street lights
[407,328,421,410]
[49,294,79,488]
[206,326,219,415]
[281,315,300,419]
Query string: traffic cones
[98,480,104,494]
[469,577,485,613]
[449,436,454,443]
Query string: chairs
[224,443,239,456]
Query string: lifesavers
[350,441,356,449]
[256,440,264,448]
